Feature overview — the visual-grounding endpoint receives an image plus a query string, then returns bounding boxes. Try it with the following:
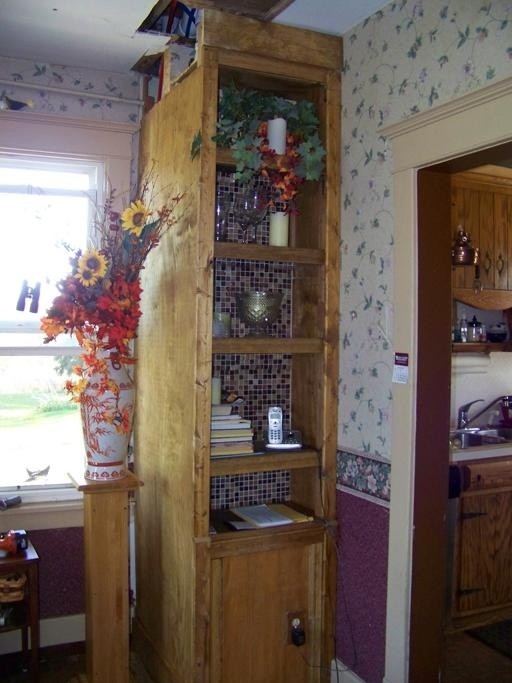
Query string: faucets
[458,395,512,427]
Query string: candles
[268,210,289,246]
[267,116,287,156]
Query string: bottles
[215,192,229,242]
[452,307,487,343]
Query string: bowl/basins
[488,333,507,343]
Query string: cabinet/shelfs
[450,165,512,353]
[449,453,512,631]
[136,9,342,683]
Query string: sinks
[449,431,508,450]
[475,429,512,441]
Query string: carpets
[464,618,512,660]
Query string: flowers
[37,161,194,434]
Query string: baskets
[0,573,27,603]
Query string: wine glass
[234,187,267,246]
[233,290,283,338]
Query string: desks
[0,533,41,683]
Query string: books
[211,500,315,534]
[210,403,263,456]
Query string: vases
[80,337,136,480]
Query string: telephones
[265,406,303,452]
